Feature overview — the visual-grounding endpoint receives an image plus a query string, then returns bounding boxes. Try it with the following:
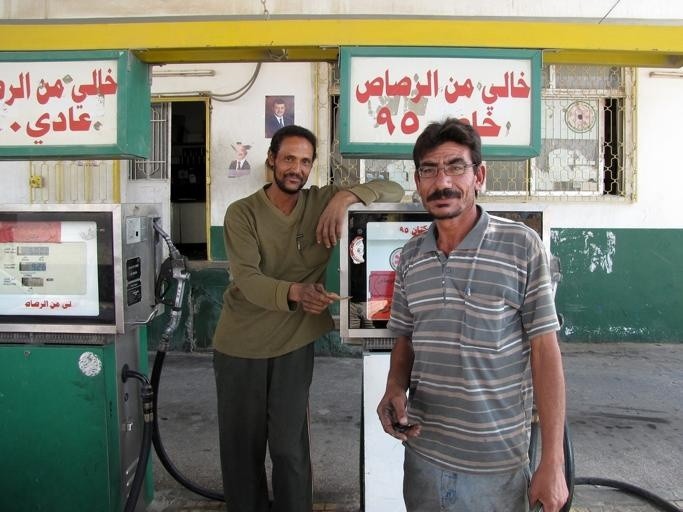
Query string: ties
[280,119,282,127]
[237,162,240,169]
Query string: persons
[375,116,570,512]
[347,214,376,329]
[212,124,405,512]
[228,142,251,169]
[265,99,294,138]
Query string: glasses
[417,162,481,178]
